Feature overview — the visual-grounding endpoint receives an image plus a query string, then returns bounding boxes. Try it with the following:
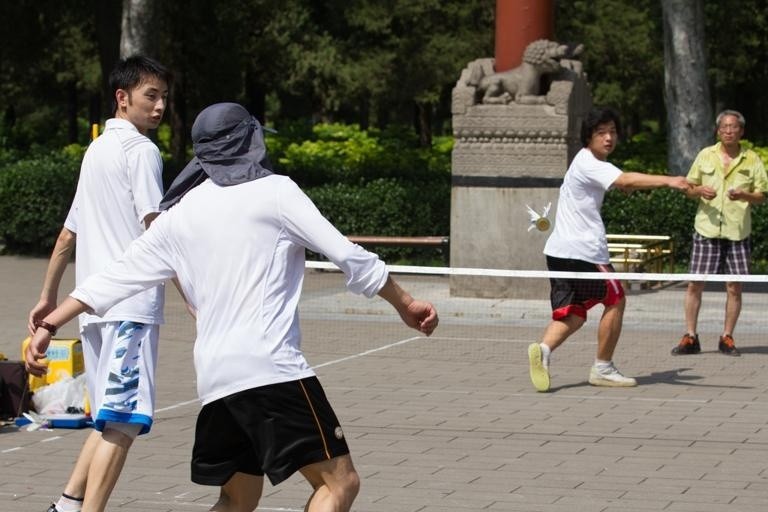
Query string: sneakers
[588,361,637,388]
[528,343,550,393]
[718,335,741,357]
[673,334,701,354]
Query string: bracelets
[35,320,57,336]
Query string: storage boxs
[21,337,85,392]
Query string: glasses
[718,123,739,132]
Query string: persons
[528,104,689,392]
[27,54,198,511]
[669,109,768,357]
[24,100,439,511]
